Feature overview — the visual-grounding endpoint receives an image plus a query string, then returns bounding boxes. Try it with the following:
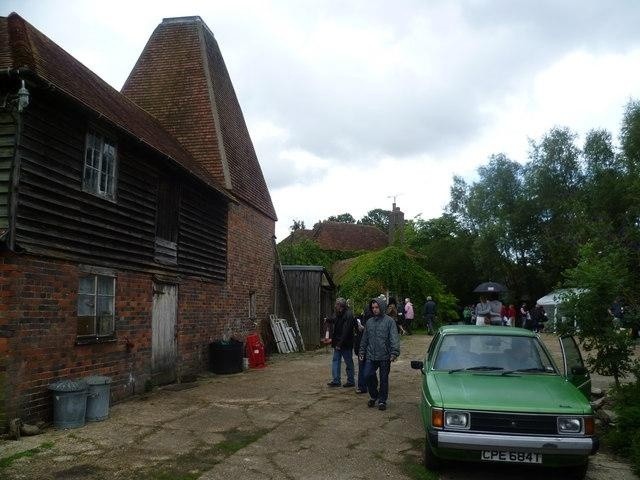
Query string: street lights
[2,76,31,115]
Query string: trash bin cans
[83,375,112,422]
[49,380,87,428]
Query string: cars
[414,324,600,480]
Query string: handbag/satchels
[484,317,491,324]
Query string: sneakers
[368,400,386,411]
[327,380,367,394]
